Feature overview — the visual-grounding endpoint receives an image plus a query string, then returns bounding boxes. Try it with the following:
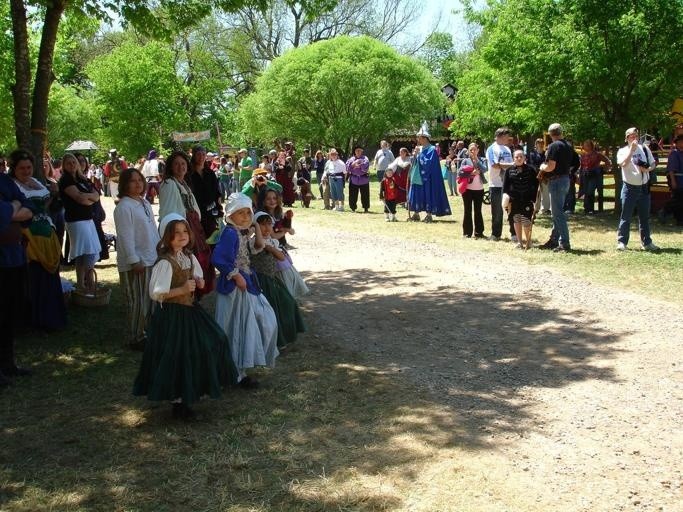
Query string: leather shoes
[9,366,31,374]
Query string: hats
[238,149,247,154]
[225,193,253,225]
[624,127,638,142]
[253,168,267,175]
[159,213,186,238]
[253,211,272,224]
[416,121,431,138]
[327,148,339,155]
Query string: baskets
[72,269,111,306]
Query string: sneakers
[465,231,502,241]
[511,235,534,250]
[239,375,260,389]
[422,215,432,223]
[323,204,345,211]
[641,242,660,252]
[407,214,420,222]
[616,241,626,250]
[385,213,397,222]
[539,245,570,252]
[172,402,188,417]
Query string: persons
[500,148,539,252]
[656,133,682,228]
[535,122,580,253]
[56,153,103,292]
[484,126,515,242]
[210,191,279,389]
[615,125,661,254]
[647,136,663,165]
[0,137,314,353]
[0,156,37,387]
[456,142,488,240]
[297,119,577,225]
[130,210,238,420]
[576,137,612,215]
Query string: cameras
[256,175,263,181]
[634,159,650,168]
